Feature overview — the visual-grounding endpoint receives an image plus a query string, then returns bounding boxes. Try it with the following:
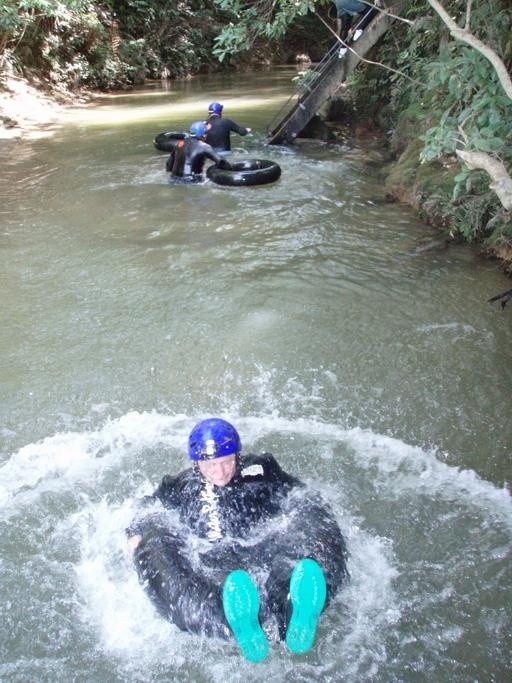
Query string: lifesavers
[134,474,345,640]
[206,159,281,186]
[154,131,188,152]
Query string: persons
[124,418,326,663]
[166,120,233,184]
[331,0,382,59]
[204,103,252,156]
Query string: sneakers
[282,557,328,655]
[221,568,270,662]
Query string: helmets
[207,101,223,117]
[189,120,211,140]
[187,417,242,461]
